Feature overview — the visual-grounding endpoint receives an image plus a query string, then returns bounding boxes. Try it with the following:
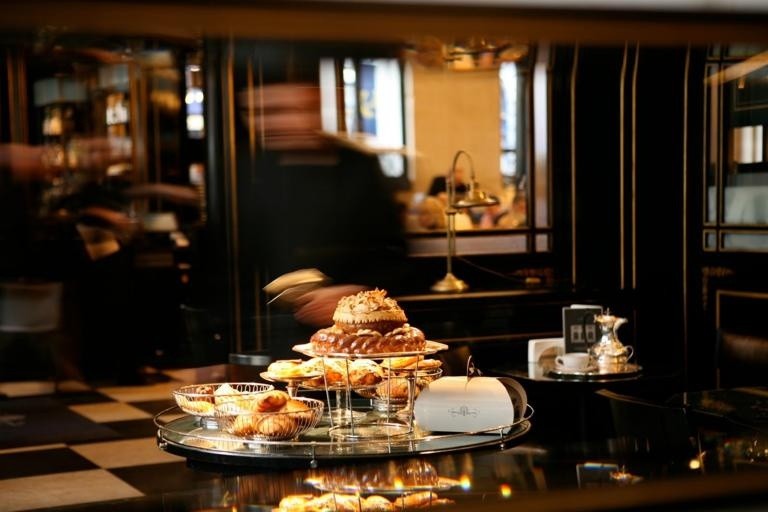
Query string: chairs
[596,385,764,479]
[0,281,64,399]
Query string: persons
[417,166,527,230]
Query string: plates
[294,342,449,357]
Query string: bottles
[588,315,634,364]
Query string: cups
[555,352,589,371]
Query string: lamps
[430,150,501,294]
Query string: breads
[271,457,455,512]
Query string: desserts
[179,286,443,441]
